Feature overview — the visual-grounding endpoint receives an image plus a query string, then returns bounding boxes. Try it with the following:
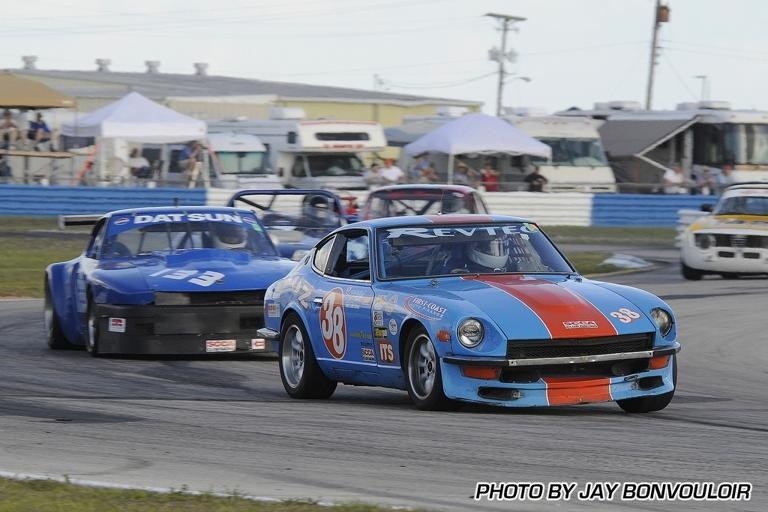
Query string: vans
[130,133,284,204]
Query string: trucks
[581,101,768,196]
[211,108,392,197]
[398,113,619,196]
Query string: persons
[129,147,151,178]
[440,197,471,214]
[708,165,738,196]
[521,164,547,192]
[0,108,21,151]
[661,159,689,194]
[361,151,502,191]
[175,139,202,174]
[440,232,519,274]
[27,111,59,152]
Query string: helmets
[439,190,467,214]
[303,195,331,220]
[466,231,510,269]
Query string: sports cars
[677,182,768,283]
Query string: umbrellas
[1,72,77,185]
